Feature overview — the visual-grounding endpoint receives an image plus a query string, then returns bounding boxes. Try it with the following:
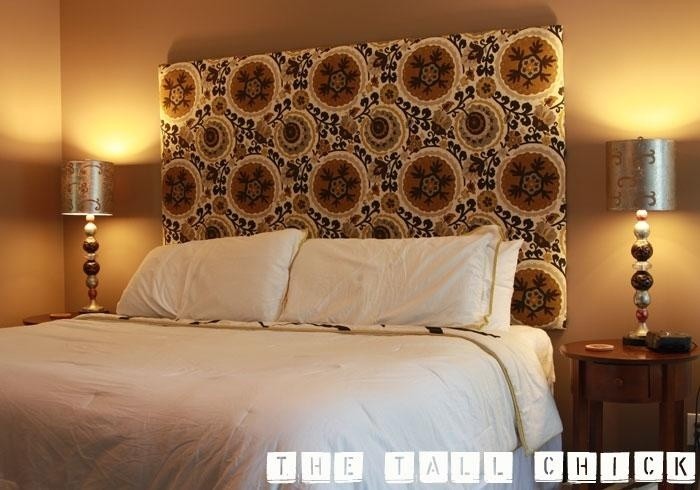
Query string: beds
[0,314,555,488]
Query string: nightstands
[559,341,700,489]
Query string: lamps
[604,139,677,347]
[58,160,114,314]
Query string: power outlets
[683,412,700,446]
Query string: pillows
[115,227,524,332]
